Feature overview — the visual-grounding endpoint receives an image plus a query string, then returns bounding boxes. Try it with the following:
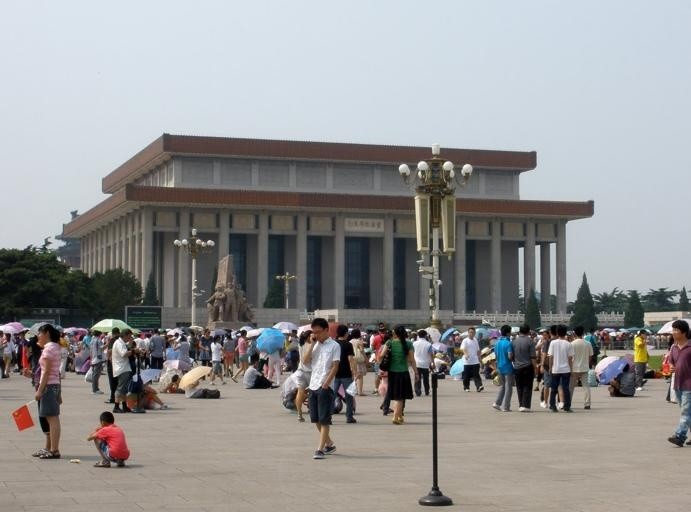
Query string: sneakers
[668,434,684,448]
[465,389,471,392]
[91,389,104,394]
[477,386,484,392]
[296,390,429,459]
[686,439,691,445]
[160,404,172,409]
[492,400,590,412]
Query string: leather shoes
[113,407,131,413]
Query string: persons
[87,413,129,467]
[667,321,691,447]
[478,323,678,413]
[34,324,61,458]
[204,269,256,322]
[460,327,483,392]
[334,321,463,423]
[32,348,50,457]
[0,319,312,421]
[304,318,336,458]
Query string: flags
[12,405,34,430]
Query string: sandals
[93,459,110,467]
[40,449,60,459]
[116,463,125,466]
[32,448,49,457]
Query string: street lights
[173,228,215,327]
[398,141,475,506]
[276,271,298,309]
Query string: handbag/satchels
[378,344,392,372]
[128,375,143,394]
[354,347,365,363]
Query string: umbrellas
[657,318,691,335]
